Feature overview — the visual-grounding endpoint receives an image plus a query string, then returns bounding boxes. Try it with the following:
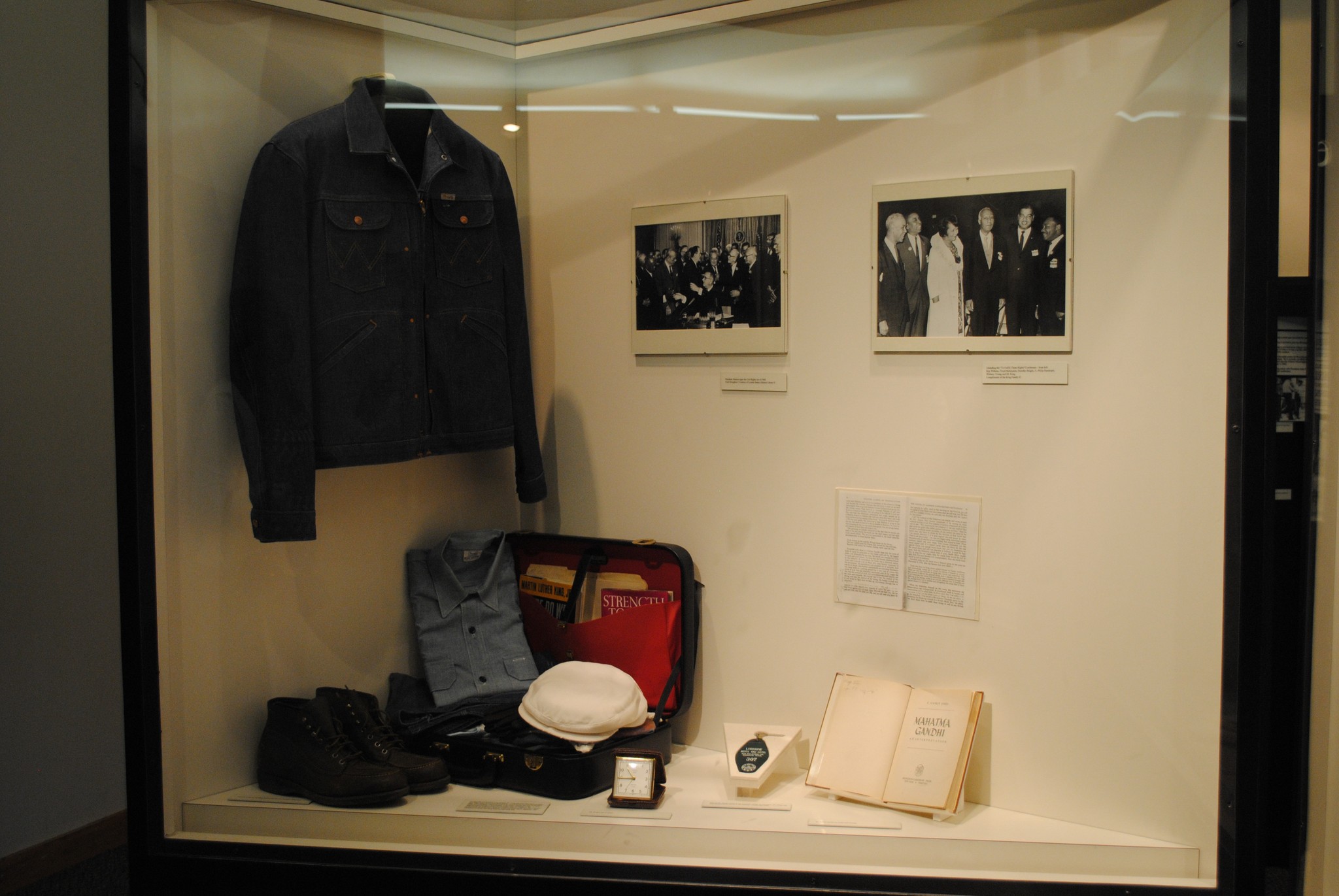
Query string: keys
[755,731,785,741]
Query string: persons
[877,203,1066,338]
[635,232,781,328]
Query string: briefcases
[402,531,705,800]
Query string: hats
[517,660,648,743]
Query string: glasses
[681,251,690,254]
[702,276,712,281]
[744,254,753,258]
[727,253,736,258]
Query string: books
[519,563,674,625]
[804,672,984,815]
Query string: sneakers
[256,697,410,805]
[315,686,451,794]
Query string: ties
[713,266,717,274]
[730,264,733,277]
[894,245,902,278]
[915,237,920,273]
[1019,231,1024,256]
[1045,242,1052,259]
[669,266,672,277]
[983,237,988,263]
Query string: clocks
[613,756,656,799]
